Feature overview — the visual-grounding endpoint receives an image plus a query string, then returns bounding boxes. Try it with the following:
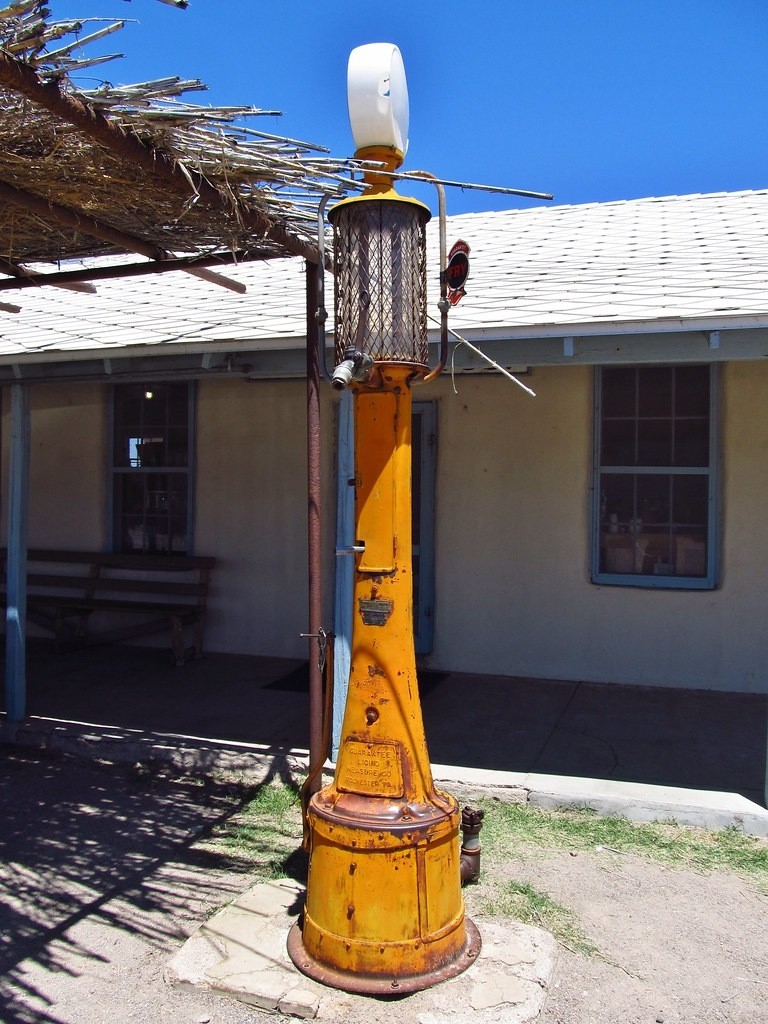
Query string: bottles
[610,513,618,534]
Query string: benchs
[0,548,216,671]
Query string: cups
[629,518,644,532]
